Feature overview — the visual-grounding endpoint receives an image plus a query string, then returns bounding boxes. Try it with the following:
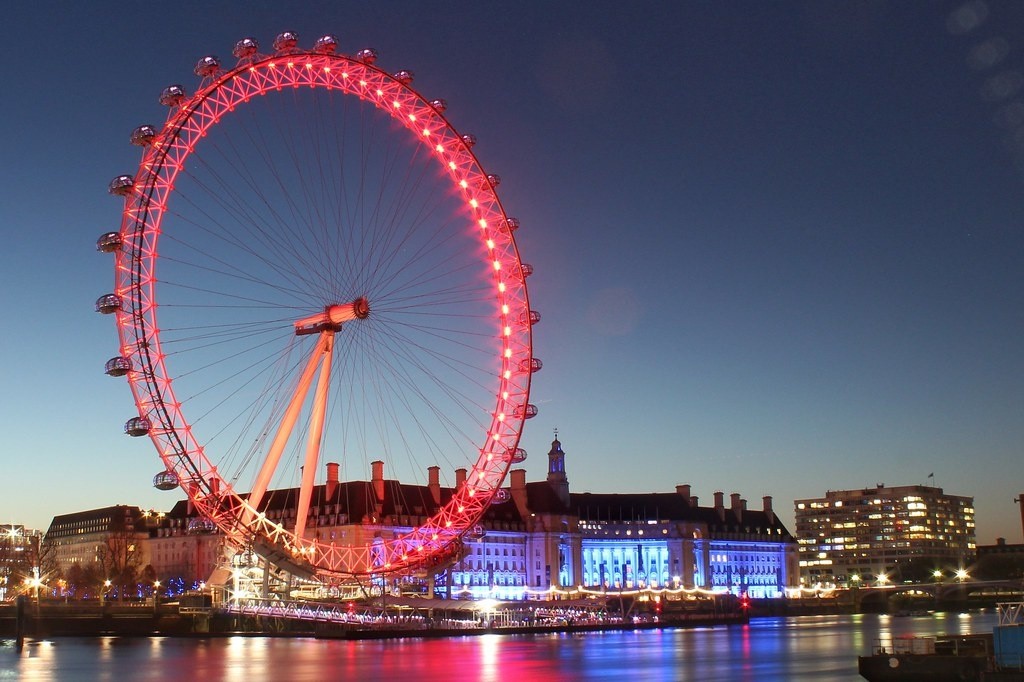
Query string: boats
[858,632,996,682]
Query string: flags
[928,472,933,478]
